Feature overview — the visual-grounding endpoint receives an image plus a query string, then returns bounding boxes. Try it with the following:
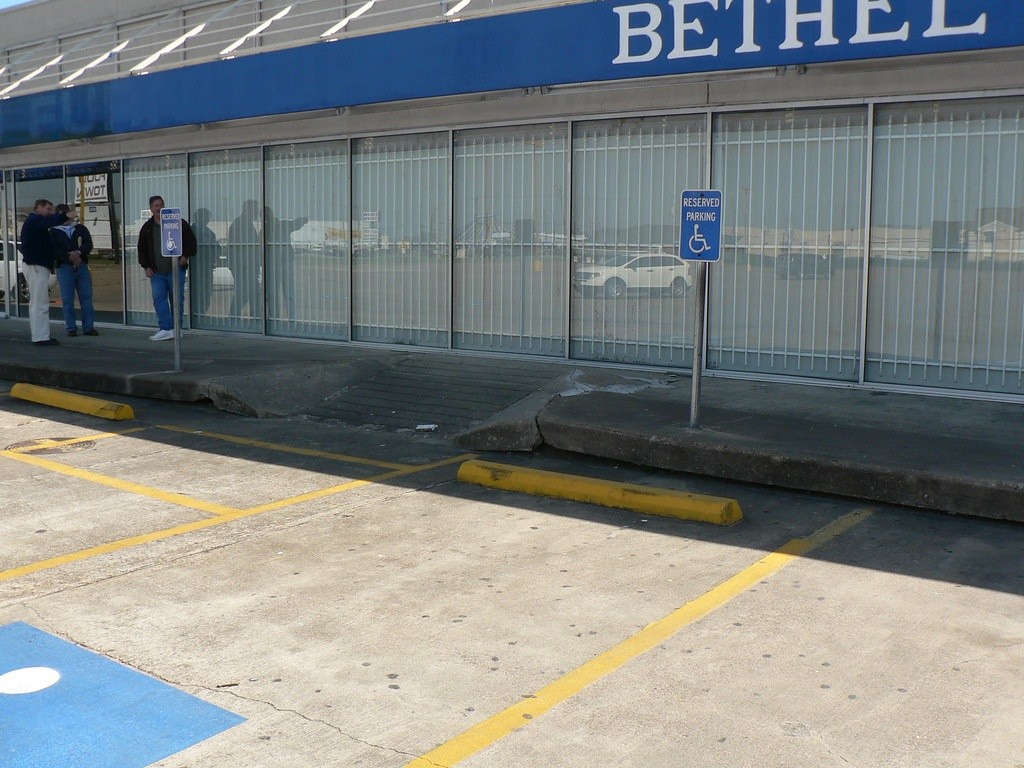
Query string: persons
[190,208,219,316]
[136,196,197,342]
[17,197,79,346]
[227,200,308,320]
[52,204,98,337]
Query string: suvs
[572,254,692,301]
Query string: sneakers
[84,329,98,336]
[149,329,175,341]
[68,330,76,336]
[32,339,59,346]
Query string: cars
[183,256,262,290]
[0,240,57,305]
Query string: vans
[775,252,835,280]
